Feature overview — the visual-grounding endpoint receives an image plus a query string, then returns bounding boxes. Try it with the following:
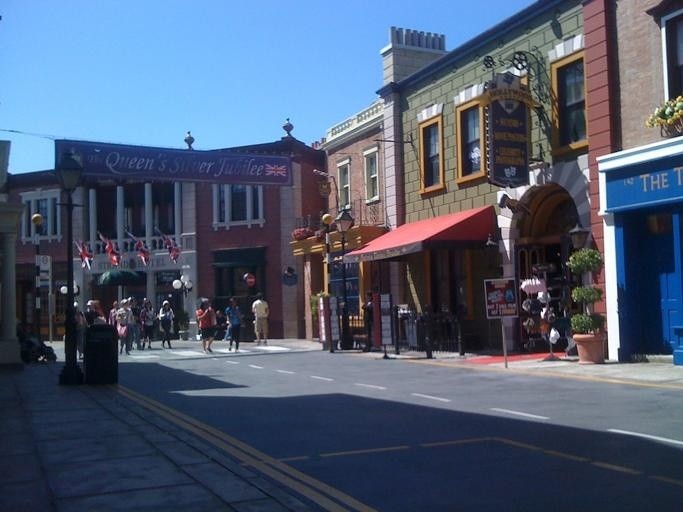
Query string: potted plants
[566,247,606,365]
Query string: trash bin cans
[82,324,118,385]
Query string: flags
[98,232,122,268]
[152,227,181,264]
[73,236,95,271]
[123,229,150,267]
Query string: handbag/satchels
[118,324,128,338]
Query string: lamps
[60,285,80,297]
[172,274,193,298]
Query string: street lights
[46,147,87,384]
[335,207,355,351]
[31,212,46,347]
[321,212,332,304]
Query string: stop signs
[247,274,255,286]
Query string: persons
[63,295,243,356]
[16,320,57,364]
[251,292,269,345]
[359,290,373,353]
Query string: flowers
[646,96,683,138]
